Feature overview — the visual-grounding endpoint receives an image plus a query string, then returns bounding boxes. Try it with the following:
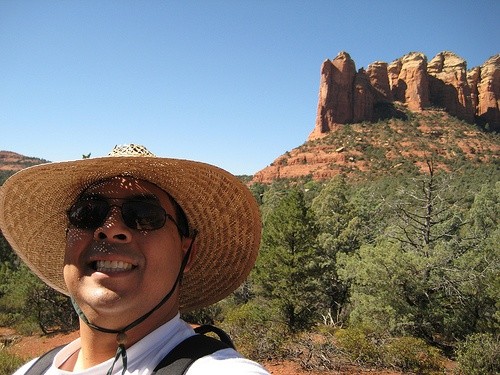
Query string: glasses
[64,198,185,232]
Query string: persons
[11,145,271,375]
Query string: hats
[0,143,261,314]
